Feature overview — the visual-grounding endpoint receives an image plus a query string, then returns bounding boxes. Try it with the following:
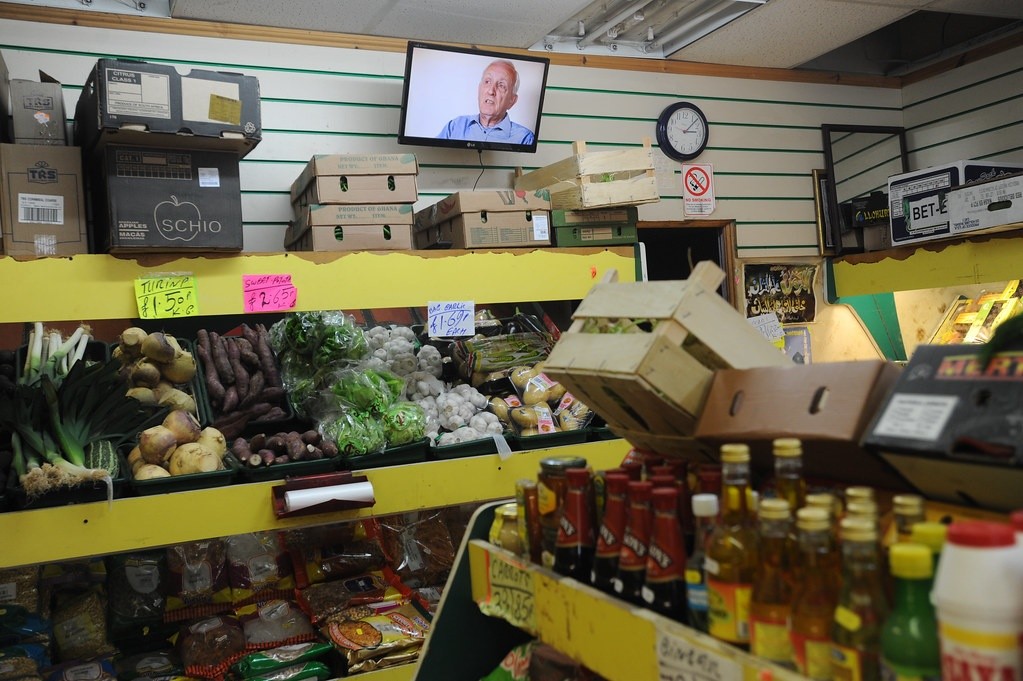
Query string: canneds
[489,457,588,568]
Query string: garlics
[358,324,504,447]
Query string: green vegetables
[265,310,427,456]
[448,333,553,386]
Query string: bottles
[486,436,1023,678]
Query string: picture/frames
[812,168,836,257]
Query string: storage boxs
[516,135,661,210]
[838,203,856,234]
[842,229,864,248]
[852,193,890,226]
[414,189,553,249]
[0,143,89,260]
[540,261,797,463]
[551,206,639,246]
[889,160,1023,247]
[871,343,1023,512]
[74,58,263,173]
[290,153,420,219]
[864,226,891,252]
[9,69,70,146]
[948,175,1023,234]
[87,142,244,252]
[284,204,416,250]
[696,358,921,496]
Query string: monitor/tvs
[397,41,550,153]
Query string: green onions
[0,320,139,491]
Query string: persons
[436,58,535,145]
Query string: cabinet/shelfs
[0,248,639,569]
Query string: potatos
[493,361,590,436]
[196,323,339,469]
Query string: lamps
[578,0,738,53]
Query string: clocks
[655,102,709,162]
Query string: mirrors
[822,124,911,255]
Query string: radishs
[110,327,226,480]
